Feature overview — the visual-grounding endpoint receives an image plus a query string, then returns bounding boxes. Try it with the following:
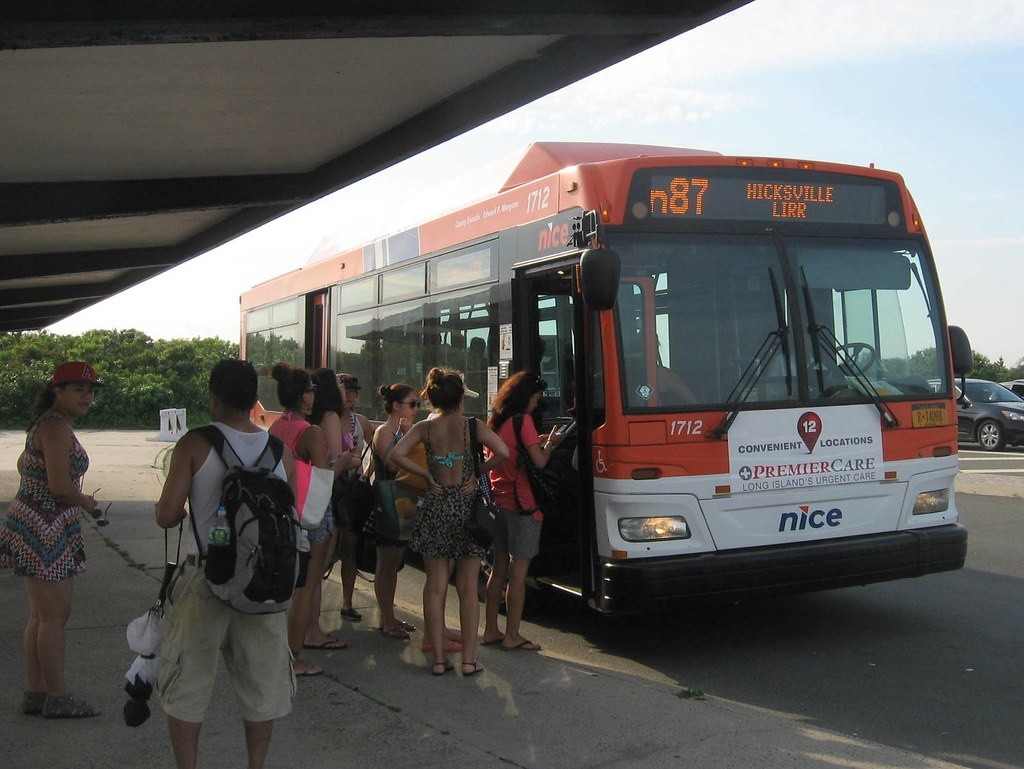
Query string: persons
[0,362,105,718]
[269,364,362,676]
[307,368,376,619]
[371,384,422,639]
[593,332,695,406]
[155,358,298,769]
[485,371,571,657]
[769,353,831,377]
[392,367,510,676]
[469,338,487,367]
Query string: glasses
[304,383,318,395]
[398,400,423,409]
[91,487,112,527]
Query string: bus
[238,141,974,621]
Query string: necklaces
[388,420,398,429]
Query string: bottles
[209,507,231,546]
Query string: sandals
[461,658,484,676]
[432,656,454,675]
[21,689,48,715]
[40,692,102,718]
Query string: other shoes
[340,607,362,620]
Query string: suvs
[926,378,1024,451]
[989,379,1024,409]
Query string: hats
[51,361,107,389]
[335,373,362,391]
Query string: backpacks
[181,424,311,615]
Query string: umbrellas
[124,562,176,727]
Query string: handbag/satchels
[512,410,575,515]
[468,498,499,546]
[345,473,378,505]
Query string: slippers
[395,620,416,632]
[421,634,464,653]
[504,639,543,651]
[300,633,349,650]
[294,662,324,677]
[480,637,506,647]
[382,626,410,639]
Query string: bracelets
[549,440,554,447]
